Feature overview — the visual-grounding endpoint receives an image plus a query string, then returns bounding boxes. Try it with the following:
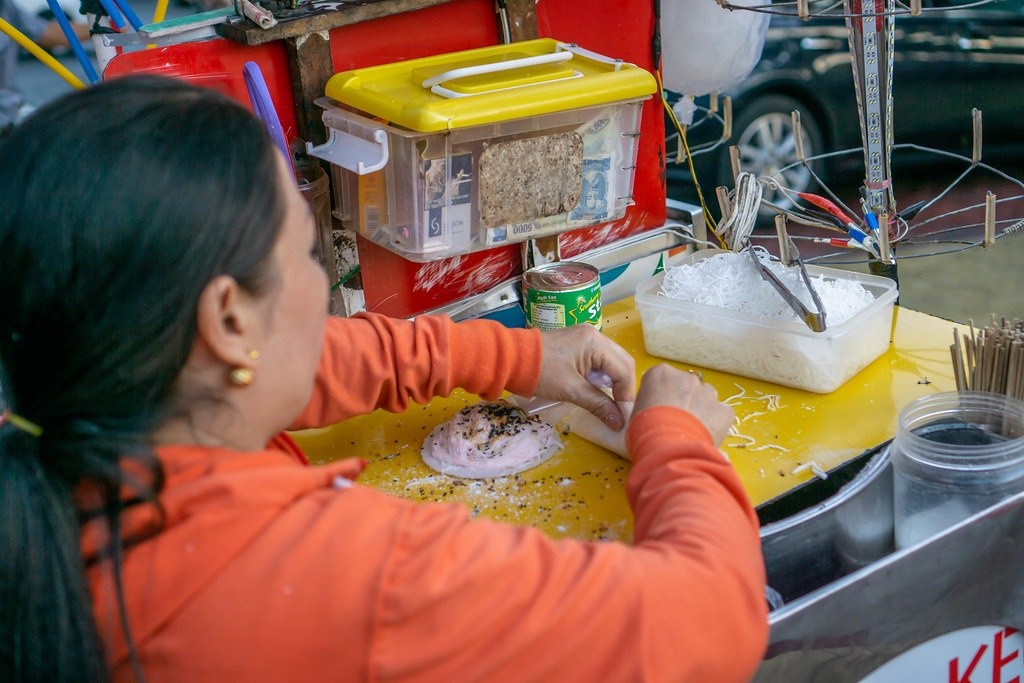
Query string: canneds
[521,260,603,333]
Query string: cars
[654,1,1023,233]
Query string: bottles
[888,390,1023,558]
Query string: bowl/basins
[634,248,900,395]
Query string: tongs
[746,233,827,334]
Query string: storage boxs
[305,38,657,263]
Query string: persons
[2,74,772,683]
